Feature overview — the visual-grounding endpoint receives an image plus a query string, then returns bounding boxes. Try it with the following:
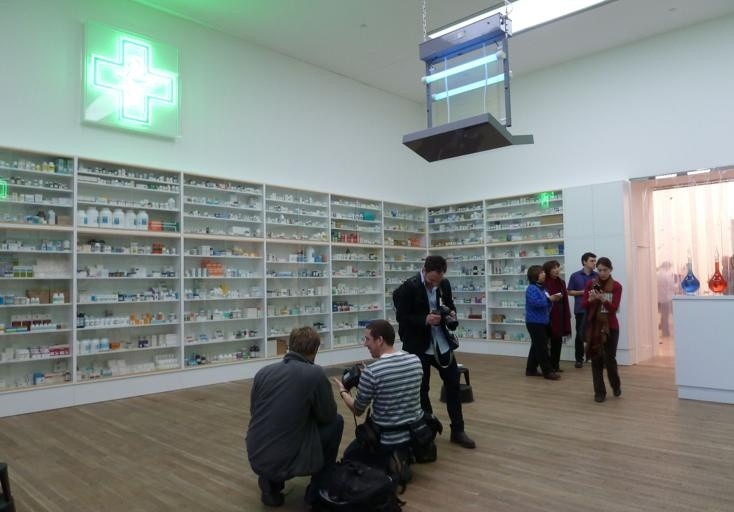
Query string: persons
[580,257,622,402]
[245,326,344,508]
[542,260,571,372]
[331,320,442,484]
[567,252,598,367]
[393,255,475,449]
[657,261,675,337]
[525,265,562,380]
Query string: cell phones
[593,284,600,293]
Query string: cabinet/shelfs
[328,192,383,351]
[564,179,635,352]
[382,198,426,343]
[181,169,265,371]
[484,191,564,344]
[73,156,182,383]
[0,147,74,395]
[263,183,330,359]
[426,201,487,341]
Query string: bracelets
[339,390,348,399]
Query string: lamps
[420,48,505,88]
[428,69,514,102]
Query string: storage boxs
[49,288,70,303]
[26,288,49,304]
[490,279,506,288]
[360,211,376,220]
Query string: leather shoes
[594,393,606,402]
[613,388,621,395]
[526,368,541,376]
[543,371,560,379]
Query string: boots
[449,420,475,449]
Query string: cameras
[342,364,361,391]
[432,305,459,331]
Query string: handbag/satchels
[311,458,405,512]
[408,418,433,451]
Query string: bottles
[680,262,699,293]
[0,147,427,387]
[708,258,727,293]
[427,191,563,345]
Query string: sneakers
[258,477,284,506]
[303,484,313,505]
[387,447,409,494]
[576,362,582,368]
